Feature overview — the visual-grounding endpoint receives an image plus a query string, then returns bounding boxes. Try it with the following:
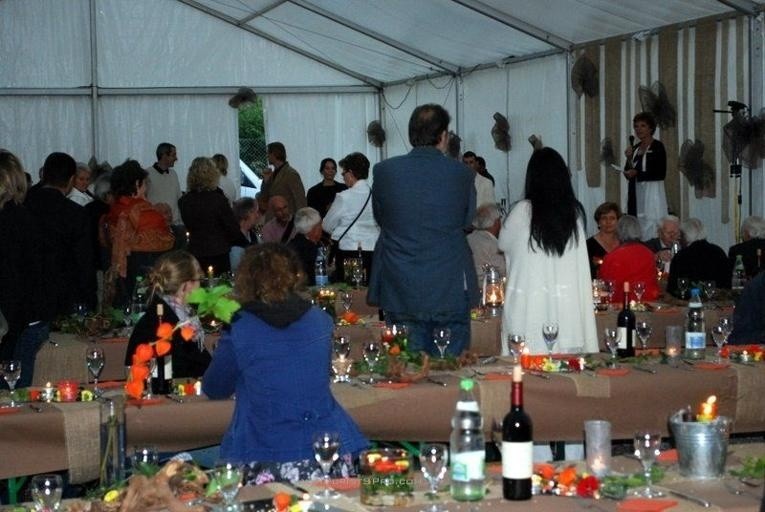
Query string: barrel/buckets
[669,411,734,477]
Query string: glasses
[341,170,349,177]
[663,231,681,241]
[190,274,207,285]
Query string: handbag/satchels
[326,238,339,265]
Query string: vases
[360,446,415,510]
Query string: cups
[584,420,614,469]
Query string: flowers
[521,353,569,372]
[367,451,409,476]
[30,379,95,402]
[536,461,601,501]
[125,285,241,398]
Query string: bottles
[450,373,487,500]
[497,357,537,498]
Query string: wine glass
[1,277,176,408]
[306,240,503,386]
[17,422,450,512]
[635,432,666,499]
[509,250,763,370]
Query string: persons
[622,110,669,249]
[72,141,381,308]
[0,139,97,391]
[587,200,764,348]
[477,156,495,185]
[370,102,476,357]
[496,146,601,357]
[202,241,371,479]
[123,250,212,381]
[457,149,502,236]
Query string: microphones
[629,135,634,159]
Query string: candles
[700,393,718,419]
